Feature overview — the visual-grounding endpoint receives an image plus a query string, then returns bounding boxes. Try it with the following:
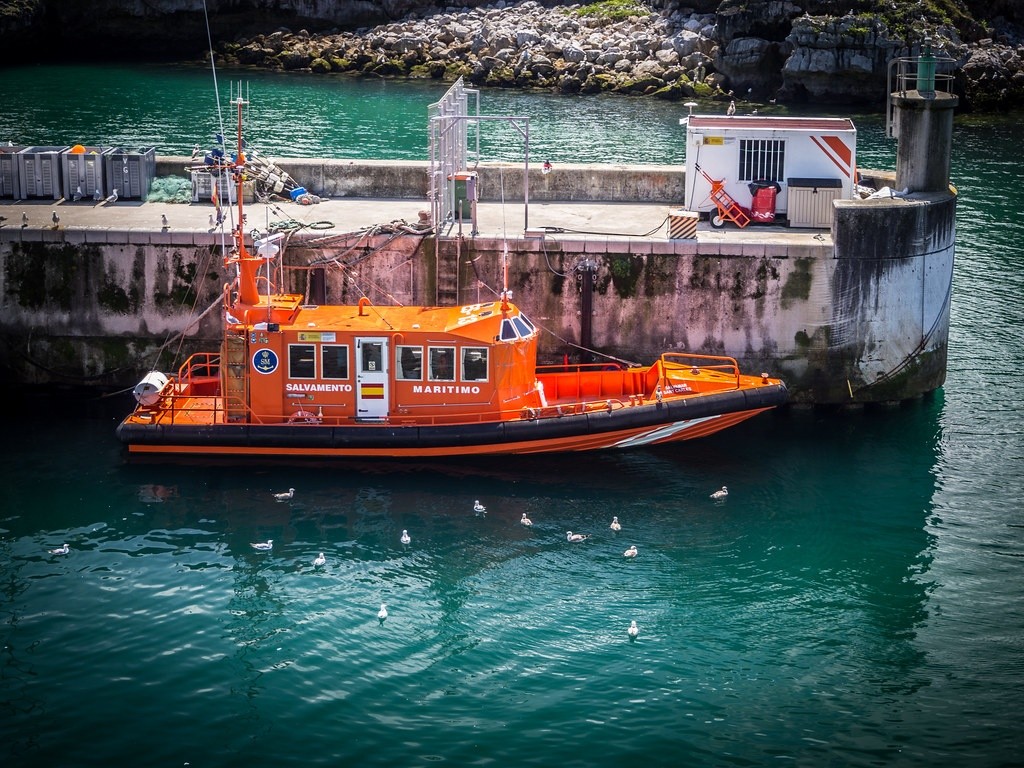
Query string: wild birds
[726,99,736,116]
[471,496,641,561]
[248,487,413,620]
[625,619,641,640]
[707,484,730,499]
[46,543,71,558]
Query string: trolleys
[694,163,750,230]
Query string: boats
[114,79,792,461]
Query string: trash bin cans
[450,170,477,219]
[62,145,113,202]
[0,144,27,200]
[753,180,778,222]
[107,145,157,198]
[19,144,70,200]
